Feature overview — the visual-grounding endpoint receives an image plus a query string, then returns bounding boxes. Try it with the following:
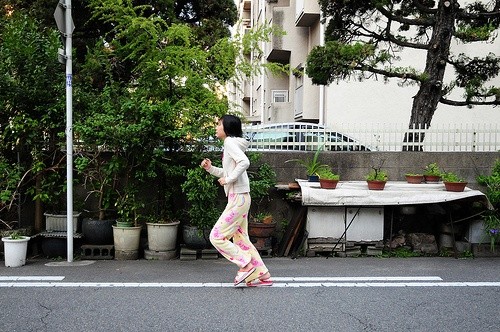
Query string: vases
[248,223,278,250]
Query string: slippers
[247,278,273,286]
[234,267,256,286]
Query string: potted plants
[319,168,340,189]
[366,169,388,190]
[182,166,220,250]
[1,230,31,267]
[443,172,468,192]
[424,163,441,182]
[145,210,180,252]
[32,187,82,232]
[284,143,328,182]
[113,193,144,227]
[405,173,423,184]
[82,179,114,245]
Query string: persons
[200,114,273,286]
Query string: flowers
[246,153,276,225]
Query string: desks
[274,182,494,257]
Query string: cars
[186,122,373,151]
